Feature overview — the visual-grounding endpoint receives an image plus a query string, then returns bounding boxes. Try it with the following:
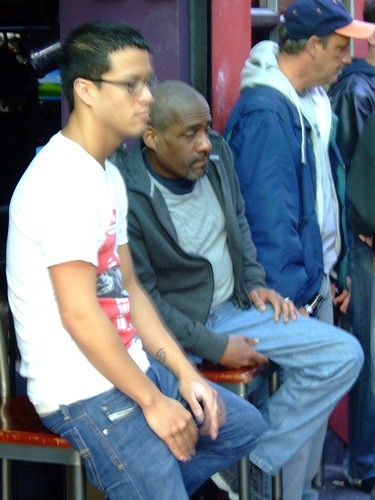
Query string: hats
[277,0,375,46]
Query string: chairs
[195,362,284,500]
[0,302,91,500]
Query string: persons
[223,2,370,495]
[7,24,267,499]
[326,3,373,499]
[113,81,364,499]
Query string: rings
[285,296,289,302]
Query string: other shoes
[211,471,240,500]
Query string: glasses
[73,75,158,95]
